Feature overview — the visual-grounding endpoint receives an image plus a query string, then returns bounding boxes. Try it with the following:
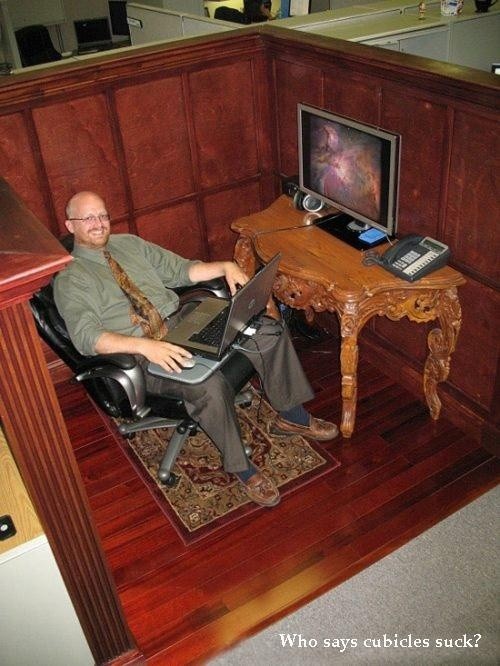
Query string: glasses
[69,214,112,223]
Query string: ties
[103,250,168,340]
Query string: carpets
[82,377,341,548]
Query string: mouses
[175,356,196,369]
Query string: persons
[52,190,341,509]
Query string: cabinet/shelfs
[404,4,500,72]
[300,12,450,65]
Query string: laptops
[158,251,283,357]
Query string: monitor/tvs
[296,101,402,252]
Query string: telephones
[374,236,450,283]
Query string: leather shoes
[269,414,339,441]
[232,458,280,507]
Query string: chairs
[26,233,256,485]
[214,6,250,25]
[203,1,244,19]
[14,24,62,68]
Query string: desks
[0,173,150,665]
[230,194,467,437]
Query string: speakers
[282,174,299,198]
